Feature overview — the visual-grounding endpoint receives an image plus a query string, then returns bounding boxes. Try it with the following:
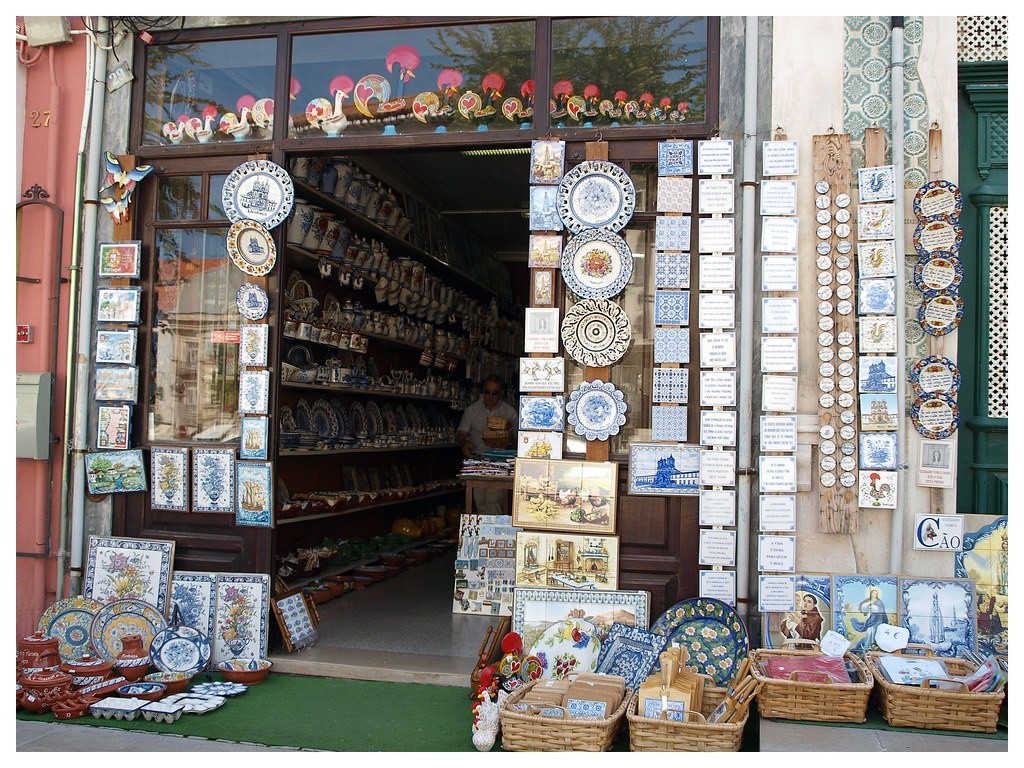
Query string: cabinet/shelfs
[202,172,524,591]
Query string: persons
[456,374,519,515]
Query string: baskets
[498,645,1005,752]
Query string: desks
[456,476,515,519]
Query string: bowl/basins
[278,477,466,517]
[143,672,194,691]
[302,528,459,605]
[279,430,354,451]
[115,682,167,702]
[218,658,274,685]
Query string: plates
[649,597,749,689]
[562,229,634,299]
[160,692,227,714]
[565,378,628,441]
[594,622,667,694]
[222,159,294,230]
[236,282,269,321]
[910,180,965,440]
[37,596,212,675]
[280,399,447,438]
[190,681,249,697]
[562,298,632,368]
[556,160,636,235]
[528,618,602,682]
[226,218,277,277]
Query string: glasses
[484,389,501,397]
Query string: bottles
[355,427,455,448]
[288,154,524,402]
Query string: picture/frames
[530,268,555,308]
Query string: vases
[301,205,329,254]
[328,220,352,264]
[317,213,340,256]
[286,199,314,248]
